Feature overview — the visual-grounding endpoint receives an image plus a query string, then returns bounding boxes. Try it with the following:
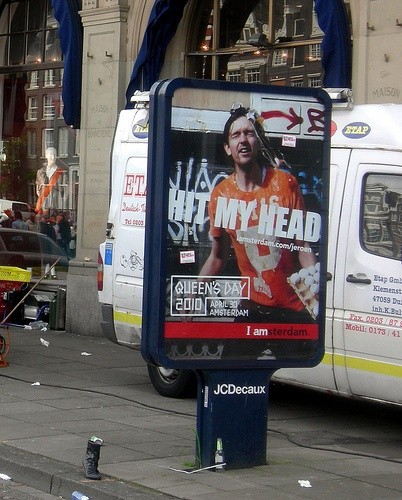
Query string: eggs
[290,262,320,318]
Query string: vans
[95,88,401,415]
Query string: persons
[36,148,69,210]
[180,107,316,358]
[1,210,71,255]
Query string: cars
[0,228,71,274]
